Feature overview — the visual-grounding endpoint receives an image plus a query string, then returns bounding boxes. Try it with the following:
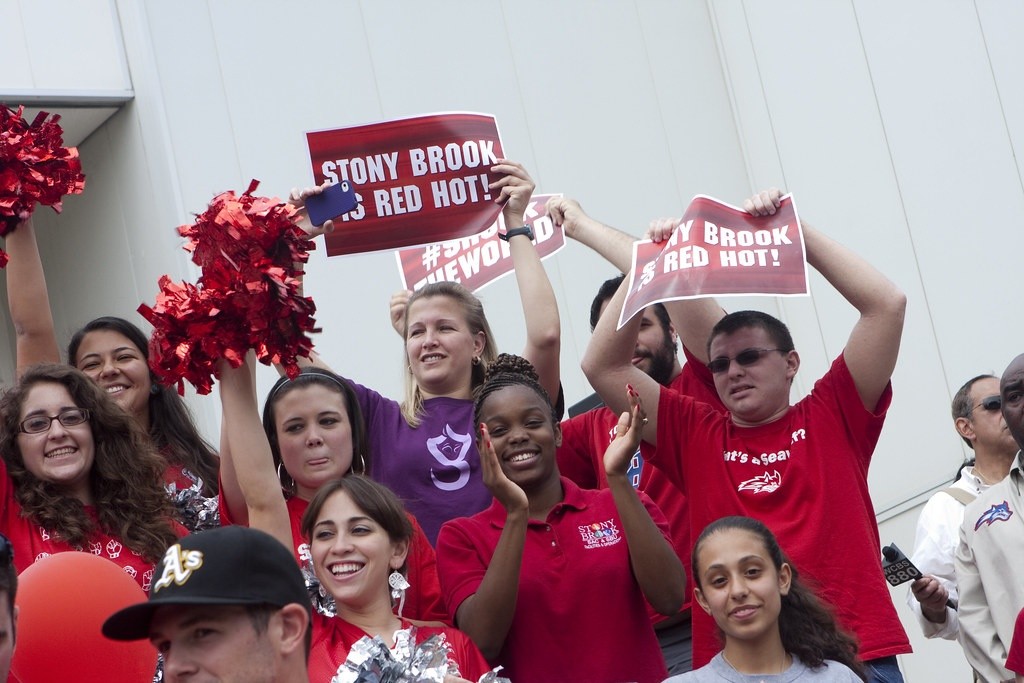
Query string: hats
[102,524,312,640]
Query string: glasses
[15,408,90,434]
[966,396,1001,416]
[706,348,789,373]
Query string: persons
[905,375,1022,640]
[0,362,192,595]
[257,159,566,547]
[955,352,1024,683]
[3,212,231,538]
[0,536,20,683]
[436,354,687,683]
[658,514,866,683]
[582,190,913,682]
[101,522,316,683]
[548,189,728,672]
[216,330,499,681]
[220,345,451,625]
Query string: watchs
[497,224,534,242]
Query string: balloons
[7,550,162,683]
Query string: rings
[643,417,648,425]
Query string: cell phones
[305,180,358,227]
